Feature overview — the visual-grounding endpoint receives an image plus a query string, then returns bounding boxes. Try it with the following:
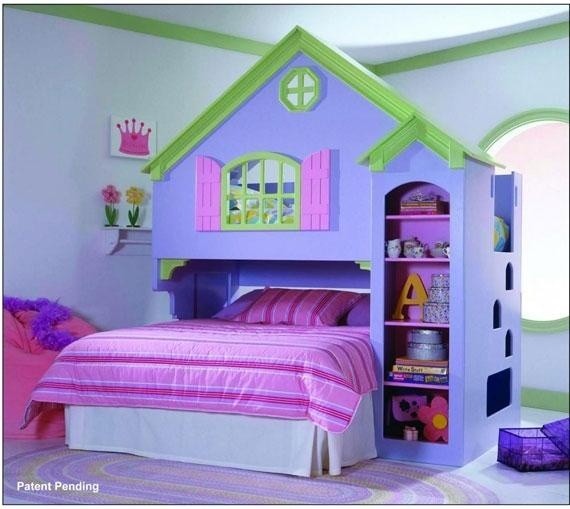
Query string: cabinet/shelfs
[370,171,521,466]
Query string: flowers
[103,185,121,226]
[126,187,143,227]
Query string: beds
[64,318,376,476]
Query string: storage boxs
[497,418,570,473]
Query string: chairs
[3,307,96,441]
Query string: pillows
[213,288,370,327]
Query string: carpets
[4,444,502,505]
